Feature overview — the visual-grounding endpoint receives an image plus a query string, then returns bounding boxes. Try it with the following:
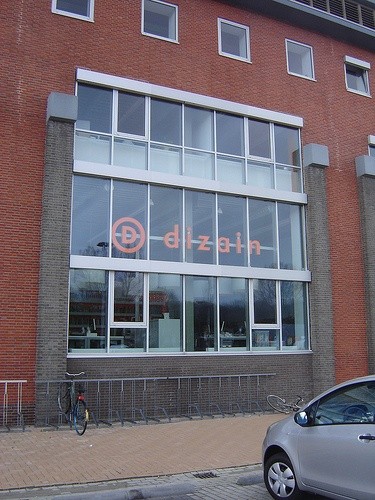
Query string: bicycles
[57,371,90,436]
[266,394,304,415]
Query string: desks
[69,334,126,350]
[198,333,247,351]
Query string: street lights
[97,241,113,348]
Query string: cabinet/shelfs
[72,280,171,347]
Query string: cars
[262,374,375,500]
